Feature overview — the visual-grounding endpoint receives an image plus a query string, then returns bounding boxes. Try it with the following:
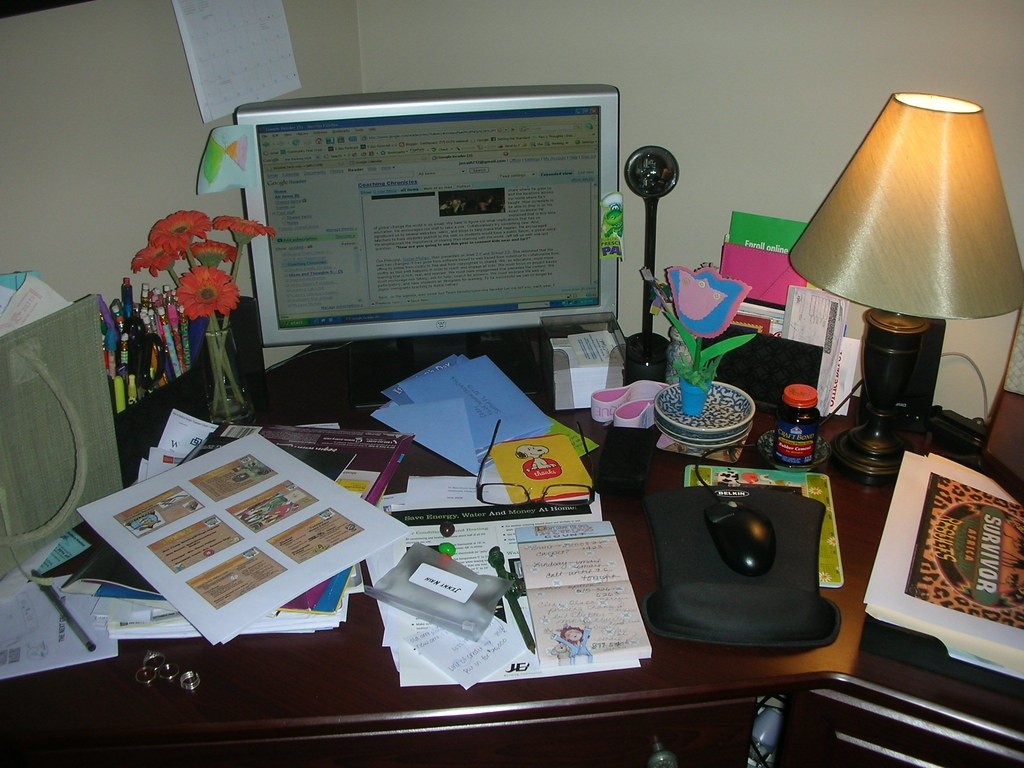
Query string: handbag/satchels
[1,294,122,586]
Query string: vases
[195,316,258,427]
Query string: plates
[654,381,756,447]
[758,430,831,472]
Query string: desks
[0,343,1023,768]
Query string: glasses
[476,419,596,506]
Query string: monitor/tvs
[238,83,618,407]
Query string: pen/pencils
[487,545,536,655]
[95,277,191,414]
[31,568,96,652]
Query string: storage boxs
[541,312,628,410]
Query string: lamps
[789,90,1024,484]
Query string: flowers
[132,209,276,418]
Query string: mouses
[705,499,778,578]
